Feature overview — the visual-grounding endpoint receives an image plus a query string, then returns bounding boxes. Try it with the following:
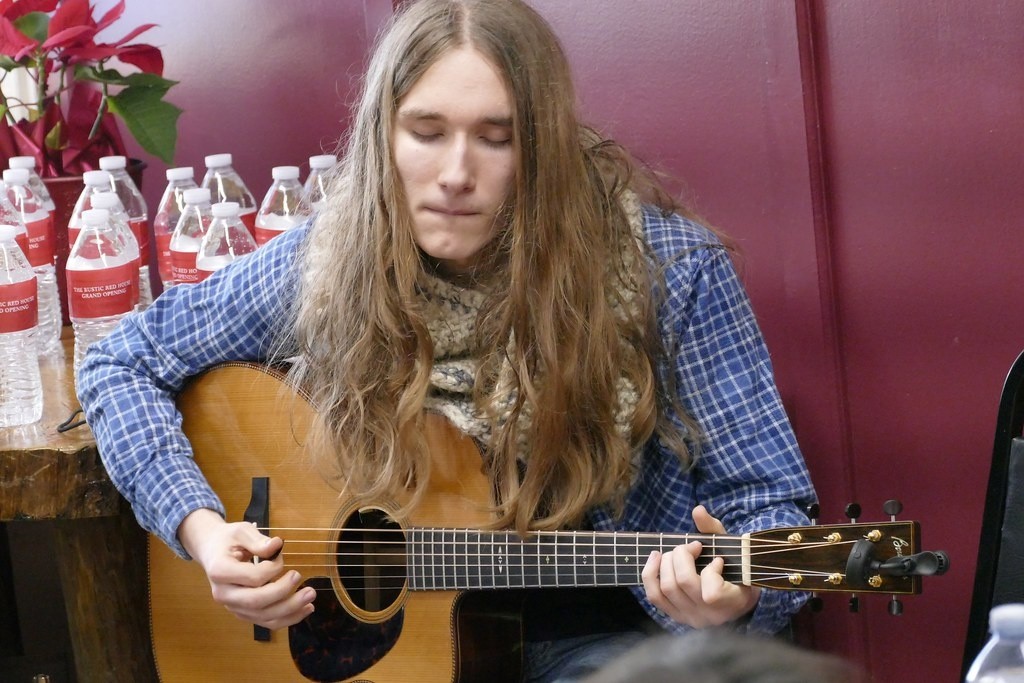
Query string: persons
[73,0,821,683]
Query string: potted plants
[1,1,184,331]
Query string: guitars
[145,359,951,683]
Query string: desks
[0,323,155,683]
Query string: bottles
[1,145,341,429]
